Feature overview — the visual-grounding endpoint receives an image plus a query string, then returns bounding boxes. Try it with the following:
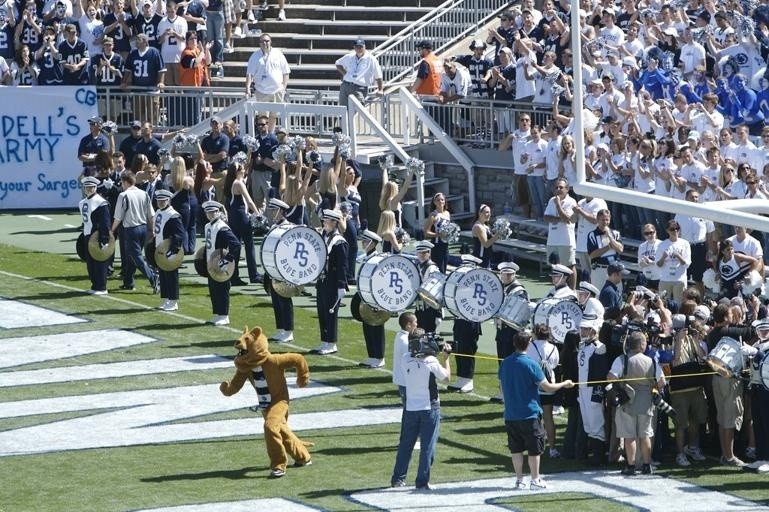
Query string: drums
[706,335,746,379]
[415,264,583,345]
[759,351,769,390]
[259,224,328,287]
[357,252,421,312]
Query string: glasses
[256,122,266,127]
[644,230,655,235]
[259,39,270,43]
[670,227,679,231]
[521,119,529,122]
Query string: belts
[596,265,606,269]
[343,80,360,87]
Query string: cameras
[703,286,730,307]
[685,314,699,337]
[646,126,656,139]
[628,284,656,299]
[649,255,656,260]
[649,290,668,310]
[604,382,629,406]
[758,293,769,304]
[745,287,761,304]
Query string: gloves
[166,250,177,260]
[337,288,347,299]
[100,243,108,249]
[435,318,442,326]
[219,260,229,271]
[103,178,114,189]
[740,342,757,357]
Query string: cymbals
[359,300,390,327]
[88,230,115,261]
[263,272,271,296]
[272,279,302,297]
[350,291,362,322]
[145,238,158,267]
[194,247,208,278]
[154,239,185,271]
[76,233,85,259]
[209,250,235,282]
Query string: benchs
[458,214,645,275]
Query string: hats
[548,264,573,277]
[154,189,174,201]
[414,240,435,253]
[752,317,769,331]
[266,198,289,210]
[605,48,618,58]
[623,56,640,70]
[497,12,513,20]
[415,40,432,49]
[443,61,453,67]
[320,208,342,223]
[210,116,222,125]
[201,201,224,213]
[81,176,100,187]
[686,129,700,143]
[88,116,103,125]
[362,229,382,242]
[354,39,365,46]
[497,261,520,274]
[602,8,616,16]
[470,38,487,51]
[692,304,710,321]
[579,280,605,334]
[499,47,511,54]
[65,24,76,32]
[46,25,56,33]
[103,37,114,45]
[131,121,142,128]
[522,8,534,18]
[591,71,614,90]
[461,254,484,267]
[672,312,686,329]
[142,0,152,7]
[608,262,631,276]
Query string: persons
[0,0,767,491]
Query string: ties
[148,184,153,197]
[116,174,119,179]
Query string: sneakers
[206,315,230,326]
[249,272,264,283]
[234,26,242,37]
[676,445,769,473]
[516,478,547,490]
[231,281,247,286]
[239,256,245,261]
[215,42,236,68]
[359,356,386,368]
[552,405,565,415]
[247,12,255,21]
[272,328,294,343]
[180,264,187,268]
[259,3,268,10]
[312,341,337,355]
[608,459,661,476]
[271,461,312,477]
[87,273,136,295]
[278,11,286,21]
[148,267,178,310]
[549,448,561,457]
[392,479,431,491]
[447,377,474,393]
[491,394,504,404]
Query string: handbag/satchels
[613,175,631,187]
[668,360,703,397]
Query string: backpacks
[533,345,555,392]
